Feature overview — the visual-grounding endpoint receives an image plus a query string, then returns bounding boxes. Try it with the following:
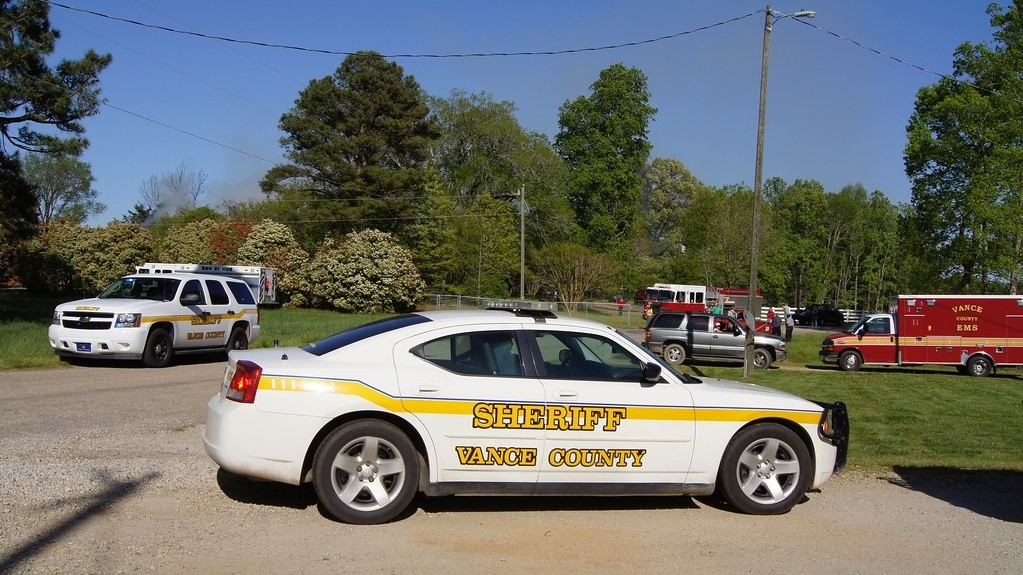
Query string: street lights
[747,12,817,375]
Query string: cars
[201,300,850,526]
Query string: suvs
[645,311,787,370]
[49,273,260,368]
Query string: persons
[794,304,818,330]
[498,332,554,377]
[645,304,653,326]
[772,313,781,337]
[766,306,776,324]
[617,296,625,316]
[783,303,790,327]
[706,302,738,333]
[785,314,794,342]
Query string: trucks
[136,262,279,309]
[819,294,1023,377]
[644,283,706,320]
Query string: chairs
[146,287,161,299]
[555,350,582,378]
[491,334,522,376]
